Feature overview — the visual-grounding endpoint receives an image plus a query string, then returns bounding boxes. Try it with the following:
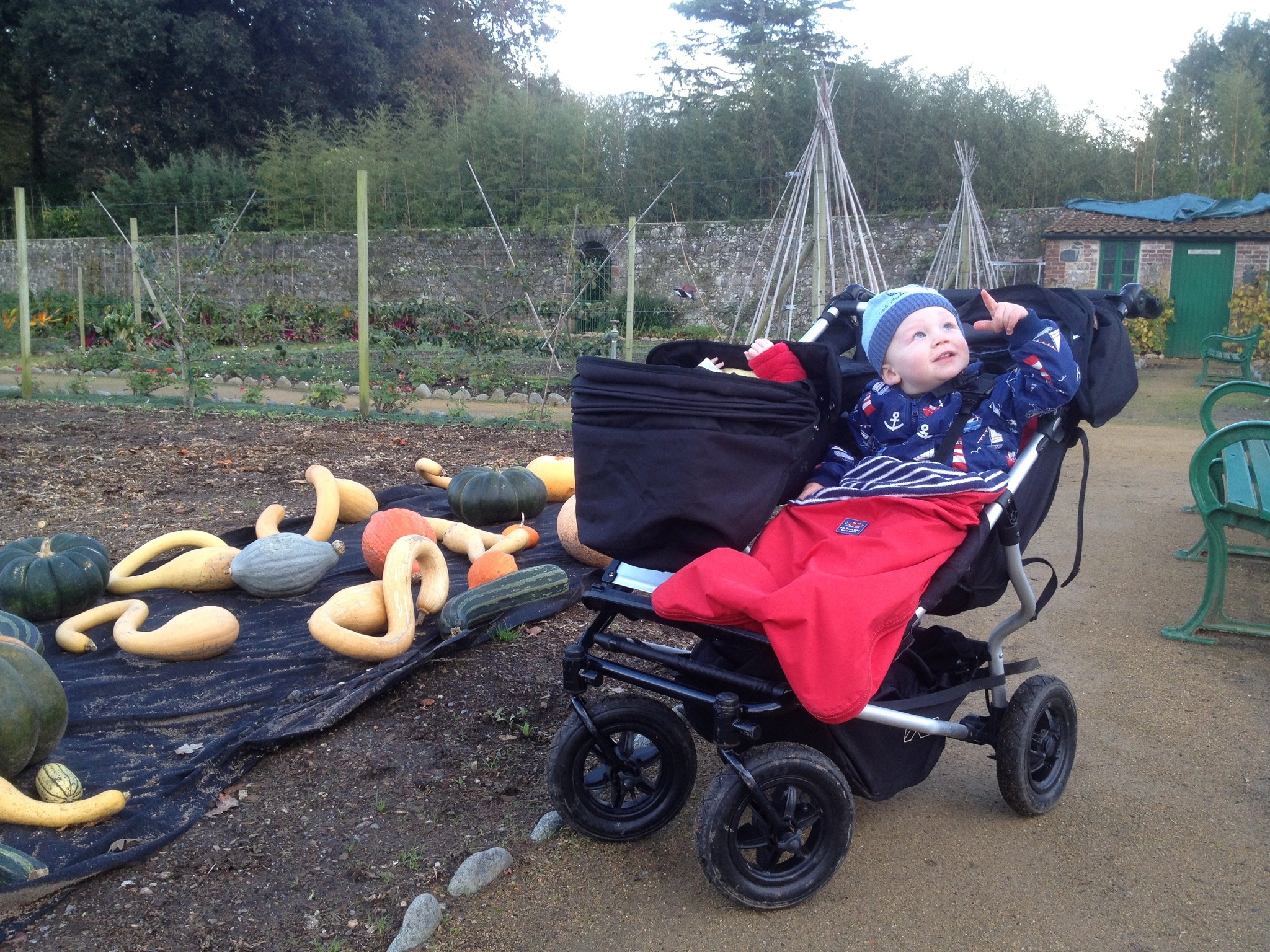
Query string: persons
[695,336,807,382]
[650,286,1080,725]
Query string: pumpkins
[0,451,613,888]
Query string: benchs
[1158,380,1270,650]
[1200,324,1265,388]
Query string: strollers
[547,283,1165,909]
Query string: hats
[861,284,966,379]
